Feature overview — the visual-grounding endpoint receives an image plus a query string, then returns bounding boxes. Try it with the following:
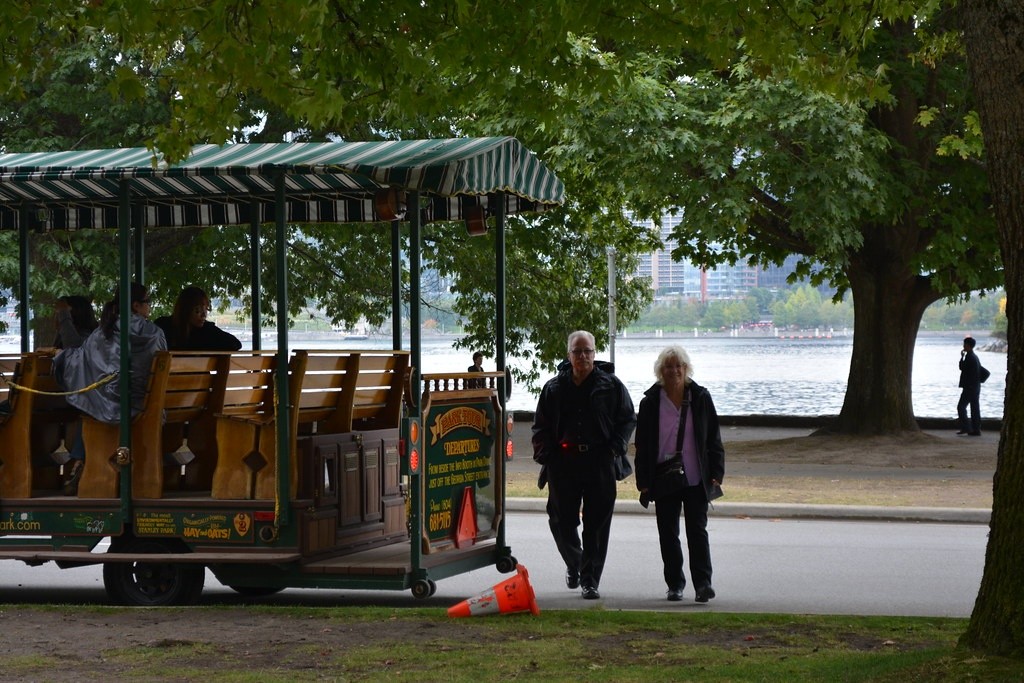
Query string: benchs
[209,347,409,497]
[0,346,78,498]
[76,345,279,497]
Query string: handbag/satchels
[980,366,990,383]
[645,452,689,508]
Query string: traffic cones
[449,564,540,618]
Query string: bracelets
[52,348,61,357]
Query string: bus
[0,135,565,604]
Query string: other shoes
[666,588,683,600]
[582,585,600,599]
[956,430,970,434]
[968,430,981,436]
[695,585,715,602]
[63,461,84,489]
[565,570,580,589]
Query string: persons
[468,352,486,389]
[154,286,242,351]
[635,345,724,602]
[956,337,981,437]
[531,331,636,600]
[35,282,168,485]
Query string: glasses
[569,349,594,354]
[138,298,151,306]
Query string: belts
[568,442,599,453]
